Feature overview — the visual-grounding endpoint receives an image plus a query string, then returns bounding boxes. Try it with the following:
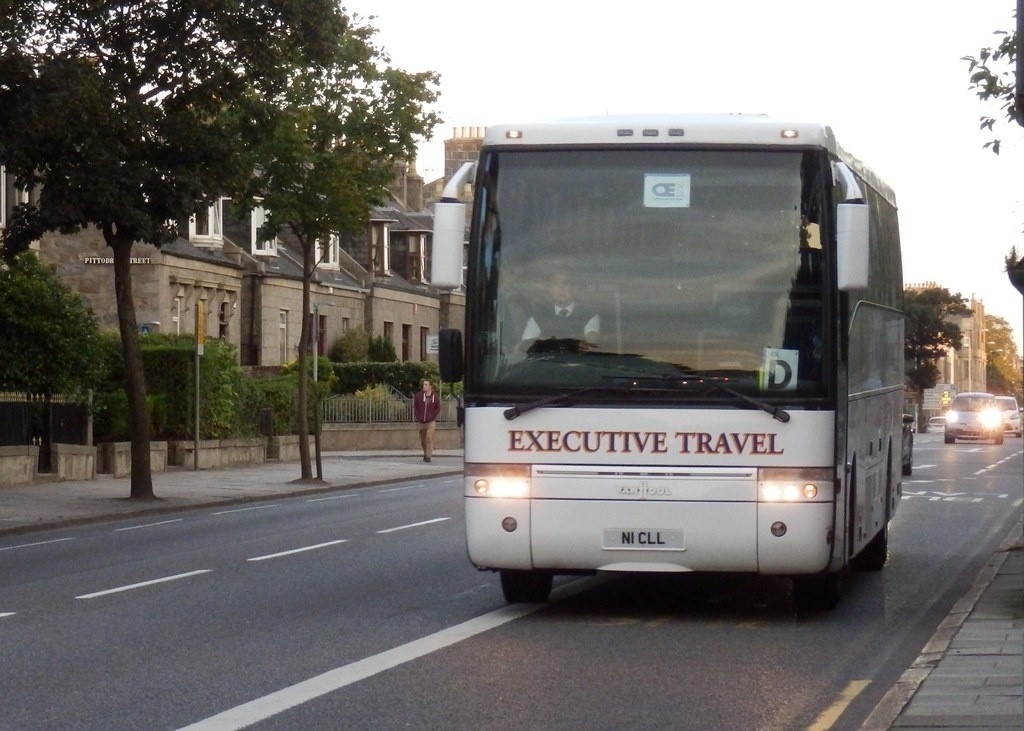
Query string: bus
[429,114,902,612]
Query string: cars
[996,396,1024,437]
[902,414,914,476]
[944,393,1004,445]
[929,416,945,428]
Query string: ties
[559,310,569,318]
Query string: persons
[415,379,441,462]
[513,265,600,355]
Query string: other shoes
[423,456,431,463]
